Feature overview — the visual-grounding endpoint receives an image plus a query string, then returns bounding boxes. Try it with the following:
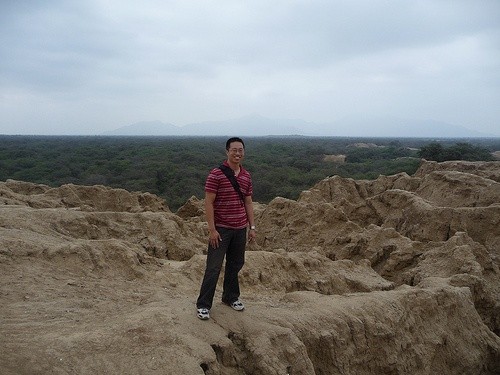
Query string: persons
[196,137,256,320]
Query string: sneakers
[196,307,210,319]
[222,299,244,311]
